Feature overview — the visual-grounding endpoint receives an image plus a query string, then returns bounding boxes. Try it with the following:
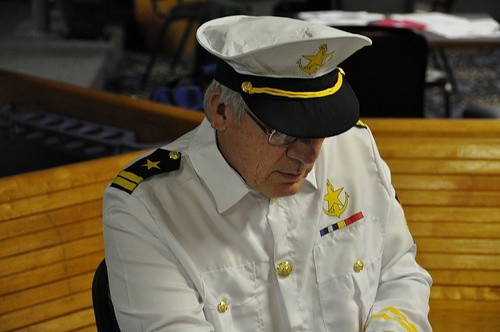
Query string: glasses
[240,103,298,145]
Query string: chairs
[141,0,244,91]
[93,258,120,332]
[325,25,430,119]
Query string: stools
[427,69,453,117]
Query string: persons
[102,15,434,332]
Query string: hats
[196,15,373,138]
